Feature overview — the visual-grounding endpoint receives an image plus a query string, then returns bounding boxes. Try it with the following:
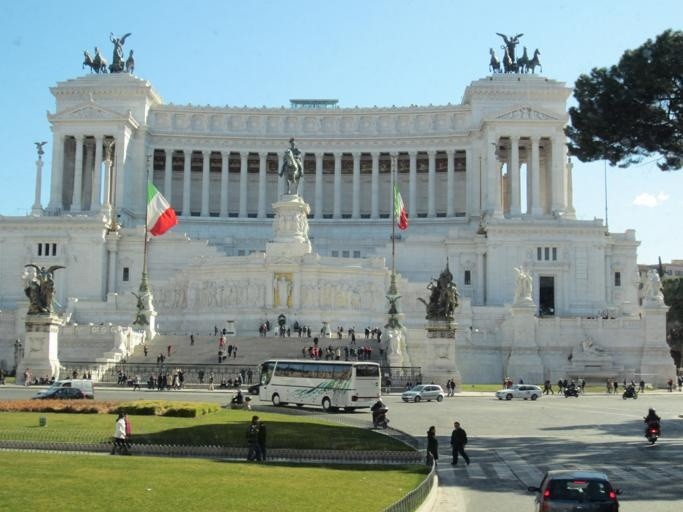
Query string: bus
[256,358,383,413]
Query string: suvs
[32,386,86,400]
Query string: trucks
[37,378,96,400]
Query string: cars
[401,383,444,403]
[495,384,545,400]
[527,470,624,512]
[247,383,261,395]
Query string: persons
[117,413,132,454]
[108,414,131,456]
[39,272,56,314]
[258,319,302,338]
[113,326,253,391]
[370,398,386,424]
[406,378,455,397]
[642,407,662,437]
[501,36,522,61]
[274,136,304,180]
[501,375,682,399]
[425,425,440,467]
[449,422,471,467]
[0,365,92,386]
[112,37,124,60]
[243,414,264,463]
[250,421,267,462]
[232,388,245,404]
[300,324,387,367]
[441,281,461,318]
[384,377,393,392]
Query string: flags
[393,185,408,229]
[146,179,178,237]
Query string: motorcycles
[367,403,390,430]
[621,385,636,400]
[640,415,663,445]
[562,386,580,398]
[230,392,251,411]
